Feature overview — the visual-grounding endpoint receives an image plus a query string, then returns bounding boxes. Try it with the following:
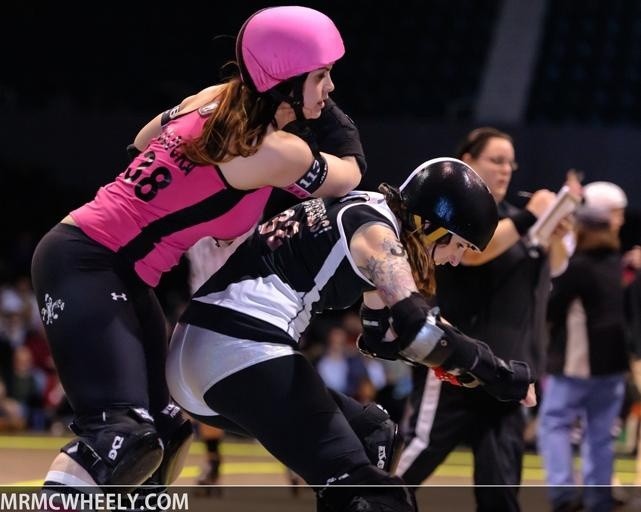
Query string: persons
[29,7,641,512]
[0,271,79,437]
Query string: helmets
[234,6,345,94]
[399,156,499,253]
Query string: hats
[583,182,628,211]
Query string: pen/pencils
[517,191,533,198]
